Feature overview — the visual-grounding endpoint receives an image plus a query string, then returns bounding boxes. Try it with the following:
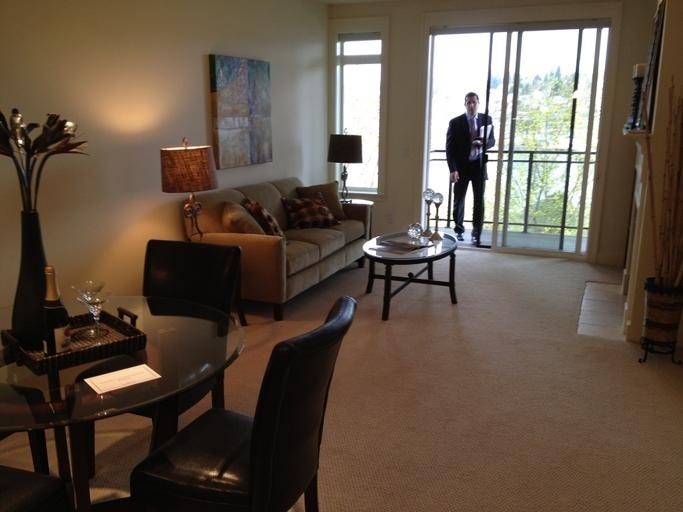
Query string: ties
[470,118,478,157]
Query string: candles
[632,63,646,79]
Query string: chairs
[2,464,65,510]
[129,294,356,509]
[75,241,245,482]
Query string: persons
[445,93,495,245]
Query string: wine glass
[70,278,111,347]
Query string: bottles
[38,265,72,358]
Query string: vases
[9,210,72,353]
[640,277,683,343]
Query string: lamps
[326,128,363,205]
[158,141,218,242]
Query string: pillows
[219,184,345,238]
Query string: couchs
[178,176,376,321]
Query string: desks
[338,198,374,240]
[3,293,246,511]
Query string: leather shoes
[472,237,480,244]
[457,234,463,240]
